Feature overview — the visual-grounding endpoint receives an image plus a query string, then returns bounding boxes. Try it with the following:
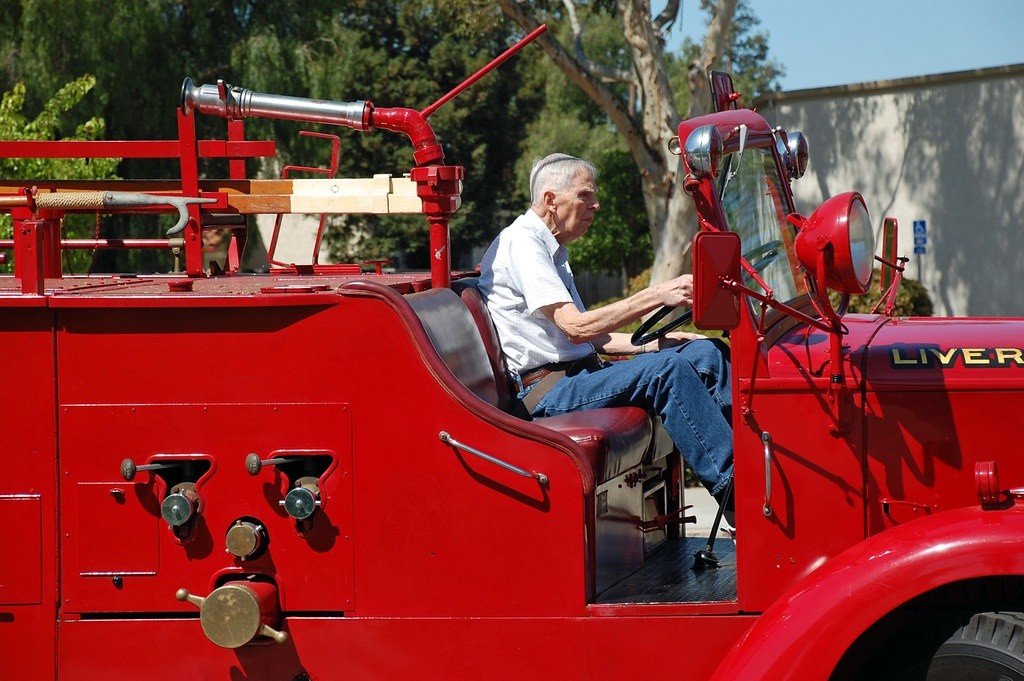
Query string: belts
[514,363,562,393]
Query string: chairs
[400,284,674,484]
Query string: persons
[477,153,736,528]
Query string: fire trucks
[0,23,1024,681]
